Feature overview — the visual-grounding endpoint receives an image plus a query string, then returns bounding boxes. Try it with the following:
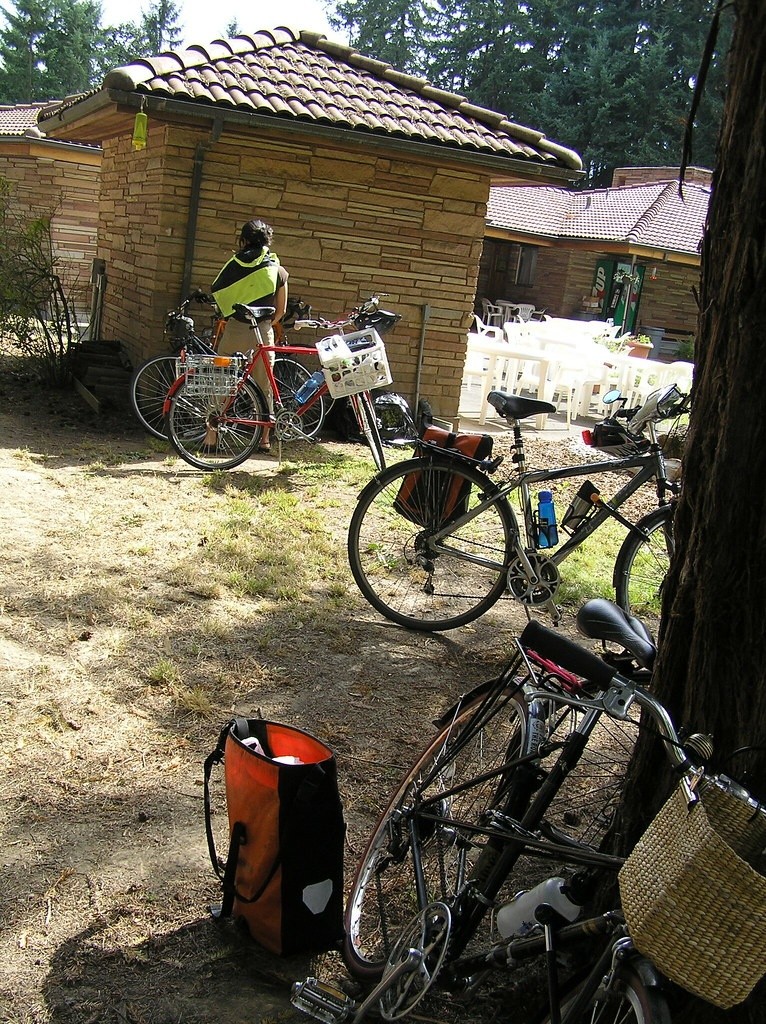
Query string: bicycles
[345,384,685,632]
[162,291,388,472]
[290,598,765,1023]
[128,289,342,445]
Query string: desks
[574,309,594,321]
[467,344,550,430]
[497,302,516,322]
[604,352,667,419]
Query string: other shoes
[199,443,219,453]
[258,447,271,455]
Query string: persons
[194,219,289,454]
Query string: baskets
[618,766,766,1010]
[315,328,393,398]
[185,355,239,396]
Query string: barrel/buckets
[638,324,666,359]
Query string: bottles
[538,491,558,546]
[295,372,325,404]
[497,872,594,940]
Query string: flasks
[562,480,600,530]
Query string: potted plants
[625,333,654,359]
[672,335,695,364]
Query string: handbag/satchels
[393,424,493,529]
[204,718,345,958]
[594,416,626,446]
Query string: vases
[622,276,635,285]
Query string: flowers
[613,272,640,285]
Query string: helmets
[354,311,403,336]
[278,301,312,328]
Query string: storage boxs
[315,327,392,399]
[580,297,602,314]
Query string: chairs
[469,298,695,431]
